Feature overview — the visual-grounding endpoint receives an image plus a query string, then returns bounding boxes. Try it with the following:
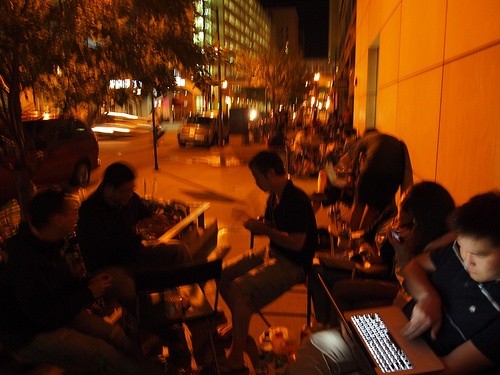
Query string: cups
[143,175,156,201]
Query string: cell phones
[390,231,401,245]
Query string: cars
[178,116,217,146]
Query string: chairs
[249,231,312,326]
[134,256,223,375]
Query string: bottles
[337,223,351,253]
[273,330,285,360]
[261,331,272,361]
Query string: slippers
[221,364,249,375]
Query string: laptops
[317,273,445,375]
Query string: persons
[351,128,405,231]
[253,109,360,206]
[401,191,500,375]
[212,150,318,375]
[77,162,194,294]
[334,181,455,302]
[0,189,136,375]
